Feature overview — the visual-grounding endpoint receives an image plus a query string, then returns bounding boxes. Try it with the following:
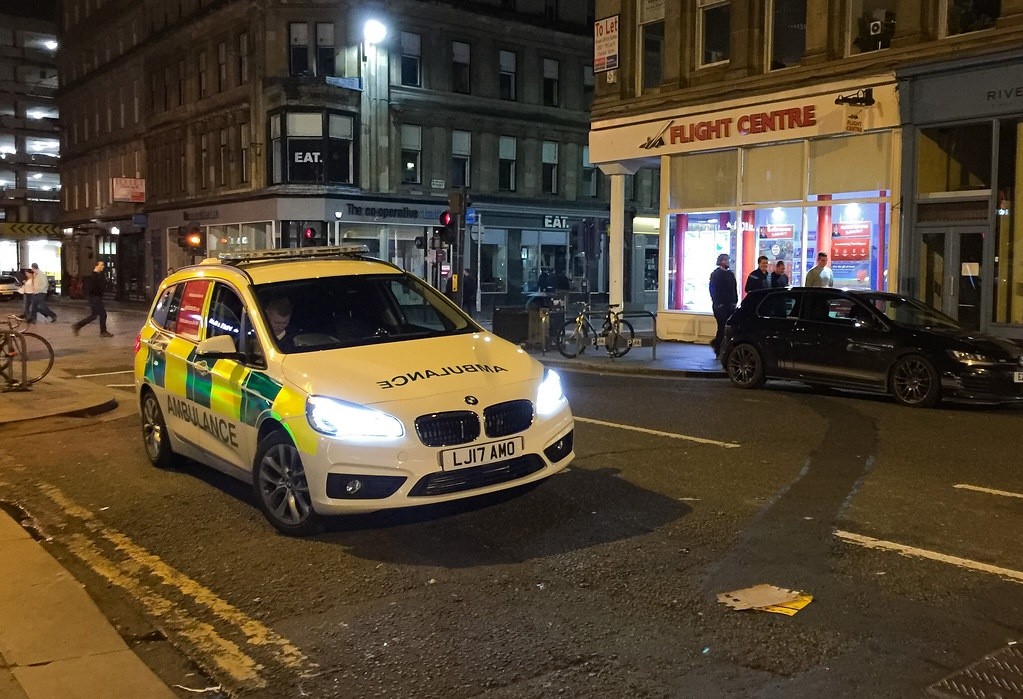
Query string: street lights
[470,214,481,312]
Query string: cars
[719,287,1023,409]
[0,275,24,300]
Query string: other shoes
[100,331,114,338]
[71,324,80,337]
[710,339,721,357]
[51,313,56,322]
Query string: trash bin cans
[529,292,564,349]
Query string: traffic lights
[302,227,316,249]
[177,222,200,252]
[439,211,457,244]
[415,237,424,249]
[446,193,462,215]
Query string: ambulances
[133,242,577,534]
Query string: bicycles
[556,301,635,358]
[0,314,55,384]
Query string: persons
[17,263,57,324]
[537,267,573,293]
[744,256,789,293]
[461,269,477,316]
[806,253,835,288]
[708,254,738,359]
[72,260,113,337]
[234,292,295,360]
[832,225,841,236]
[760,228,768,239]
